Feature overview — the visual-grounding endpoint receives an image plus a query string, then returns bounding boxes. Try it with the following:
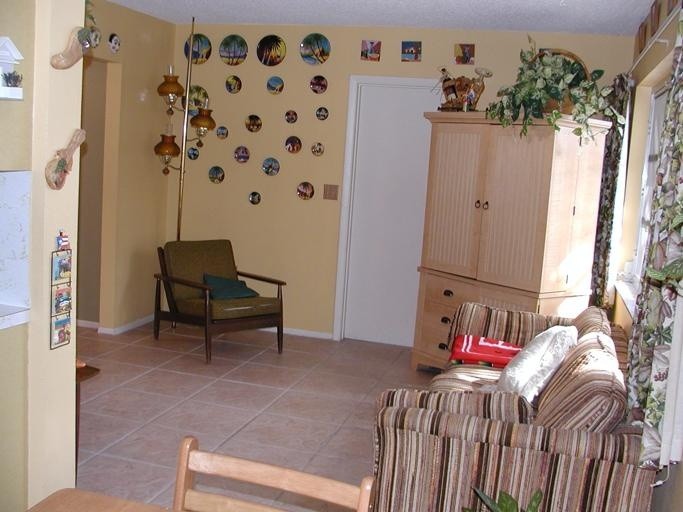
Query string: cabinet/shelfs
[410,268,540,384]
[420,111,613,295]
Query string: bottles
[441,67,457,102]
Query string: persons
[108,33,120,53]
[89,26,102,48]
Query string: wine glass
[466,67,493,105]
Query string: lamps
[152,16,217,241]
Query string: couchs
[369,304,660,512]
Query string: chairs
[152,238,289,363]
[170,434,373,512]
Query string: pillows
[202,272,260,300]
[477,322,578,401]
